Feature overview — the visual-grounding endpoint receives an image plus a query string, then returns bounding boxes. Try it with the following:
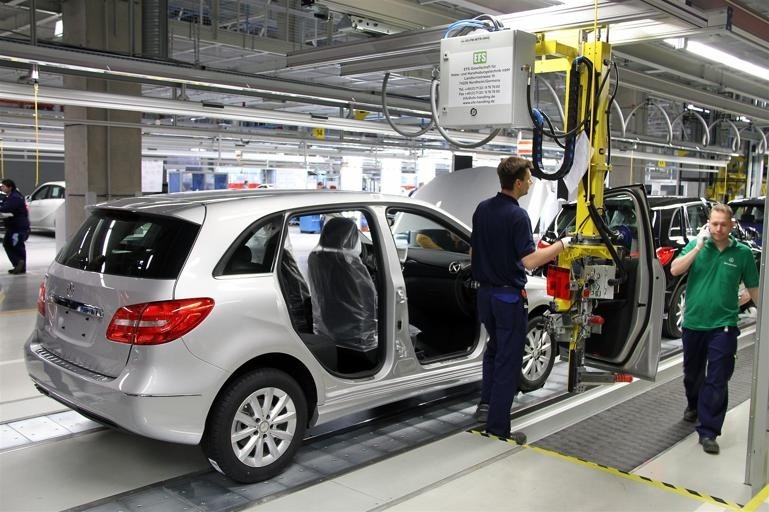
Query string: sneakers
[490,431,529,448]
[697,429,720,456]
[681,399,698,425]
[8,263,29,275]
[472,399,491,425]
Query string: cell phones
[705,226,710,240]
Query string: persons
[0,179,31,273]
[469,157,572,444]
[670,204,759,453]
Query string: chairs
[224,243,341,376]
[308,216,422,373]
[252,213,314,332]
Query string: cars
[722,193,766,248]
[22,180,66,236]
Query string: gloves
[694,222,712,250]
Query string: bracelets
[695,246,700,252]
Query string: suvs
[19,156,568,488]
[533,195,762,341]
